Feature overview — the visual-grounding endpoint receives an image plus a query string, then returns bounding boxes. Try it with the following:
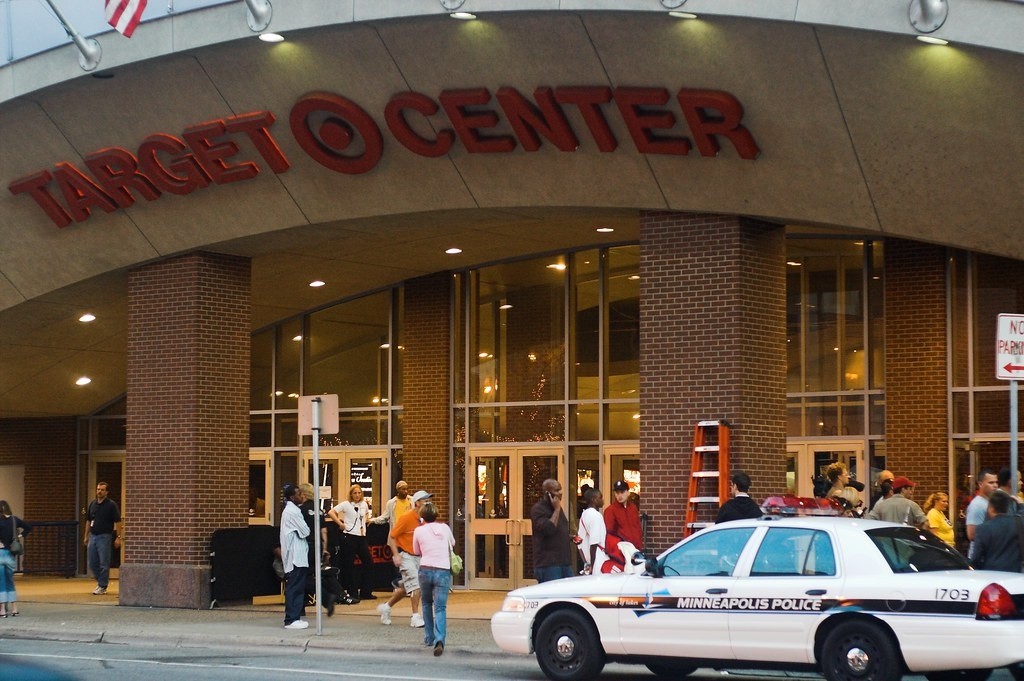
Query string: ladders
[683,419,730,540]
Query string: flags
[105,0,147,39]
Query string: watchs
[116,535,120,538]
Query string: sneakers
[409,616,425,628]
[377,603,392,625]
[285,619,309,630]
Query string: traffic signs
[996,314,1024,380]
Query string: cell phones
[546,491,553,500]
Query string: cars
[489,496,1024,681]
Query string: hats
[892,477,916,491]
[612,481,628,490]
[412,490,433,502]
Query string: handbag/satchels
[445,525,463,576]
[10,516,24,555]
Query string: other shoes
[434,642,443,656]
[11,612,19,617]
[358,594,377,599]
[92,585,106,594]
[0,614,7,618]
[340,594,360,604]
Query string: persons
[412,504,456,656]
[328,485,378,599]
[964,467,1024,574]
[604,480,643,552]
[368,480,413,597]
[922,492,956,548]
[271,482,360,630]
[868,476,930,530]
[715,472,763,525]
[530,479,577,583]
[873,469,896,510]
[0,500,32,618]
[825,461,849,499]
[378,490,433,627]
[578,488,607,576]
[83,482,120,594]
